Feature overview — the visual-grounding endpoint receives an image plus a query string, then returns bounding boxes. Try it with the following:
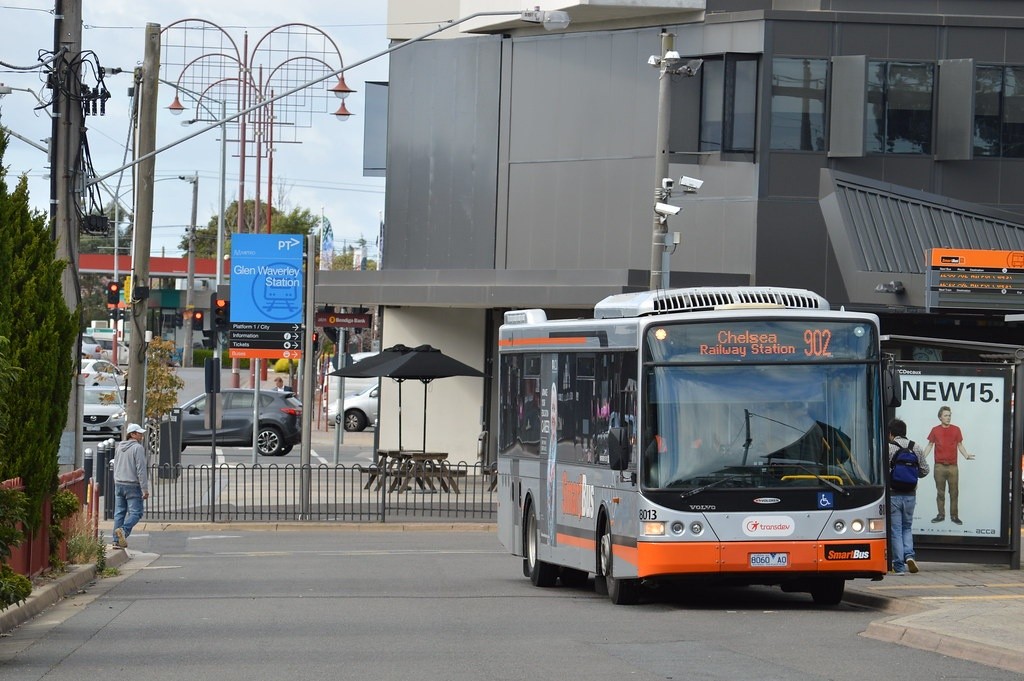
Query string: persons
[646,403,704,455]
[922,406,976,524]
[887,419,930,573]
[112,423,149,549]
[272,377,293,391]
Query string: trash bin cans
[159,408,183,478]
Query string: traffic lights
[192,309,205,330]
[108,282,119,304]
[201,329,216,347]
[209,292,231,334]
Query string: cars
[82,385,127,442]
[322,385,378,434]
[81,333,129,405]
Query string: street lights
[153,18,357,382]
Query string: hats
[127,423,146,434]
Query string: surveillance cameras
[661,178,674,189]
[679,176,703,189]
[655,202,682,215]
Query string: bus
[496,284,902,607]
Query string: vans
[320,351,380,409]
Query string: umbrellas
[326,343,490,454]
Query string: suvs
[149,386,306,457]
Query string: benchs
[362,468,467,495]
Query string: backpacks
[889,440,918,492]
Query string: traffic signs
[229,340,302,349]
[230,332,301,341]
[228,348,302,361]
[231,322,302,333]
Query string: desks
[365,450,460,493]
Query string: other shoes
[906,558,918,573]
[113,542,123,549]
[931,517,944,523]
[951,516,962,525]
[887,569,905,575]
[116,528,128,547]
